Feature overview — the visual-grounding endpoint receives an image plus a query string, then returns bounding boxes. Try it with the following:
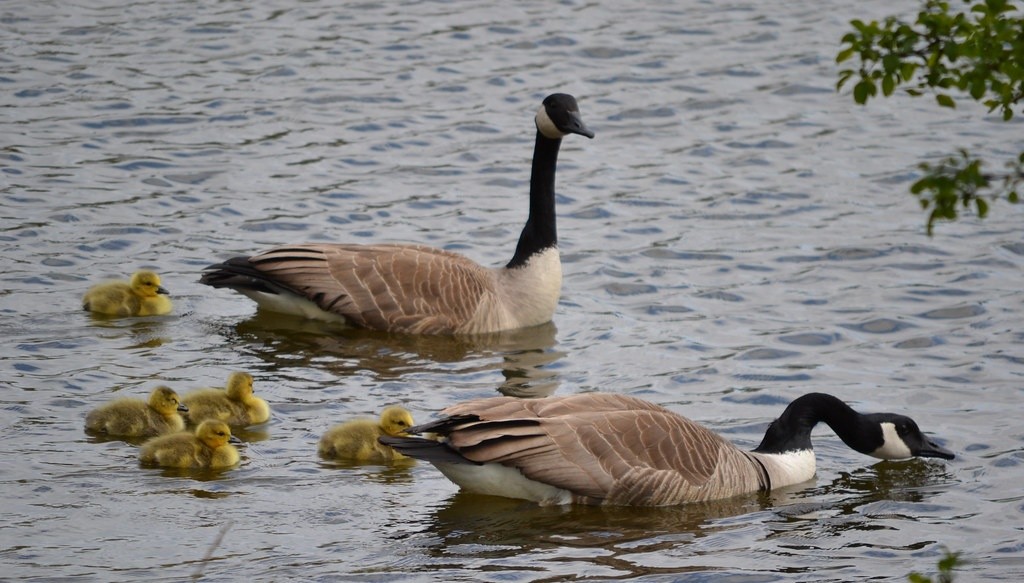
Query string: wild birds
[376,390,956,510]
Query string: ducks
[317,406,427,464]
[80,271,173,317]
[83,371,271,469]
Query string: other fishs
[194,93,597,340]
